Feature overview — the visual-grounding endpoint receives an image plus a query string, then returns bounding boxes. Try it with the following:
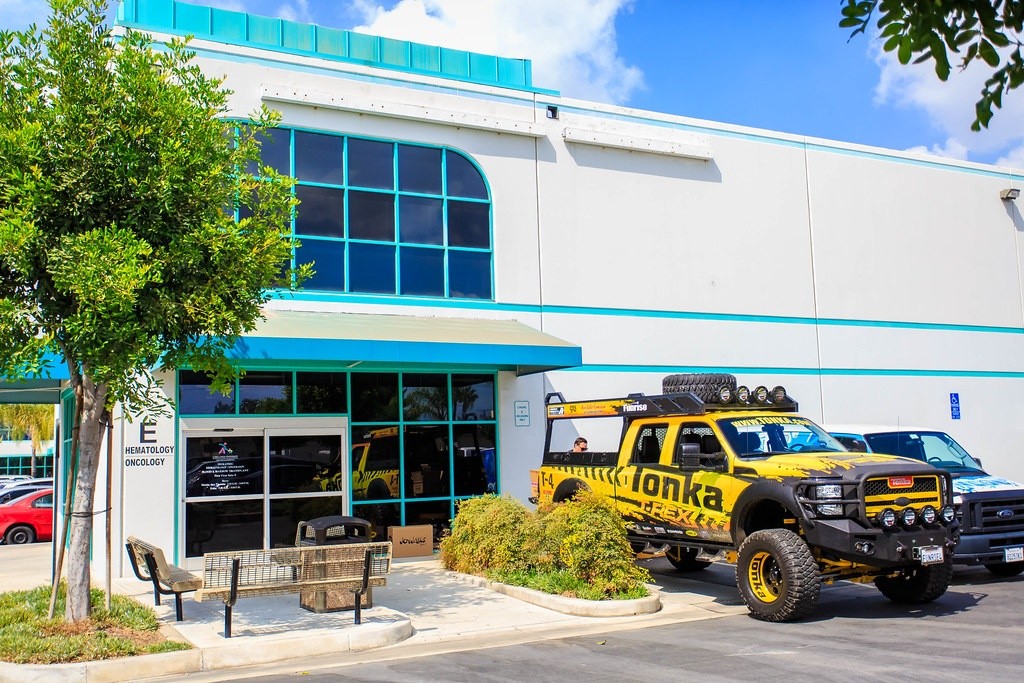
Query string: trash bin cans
[297,515,373,615]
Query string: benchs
[125,534,203,621]
[193,541,393,638]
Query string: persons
[567,437,588,452]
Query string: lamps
[1000,188,1020,199]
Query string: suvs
[300,426,484,530]
[528,373,961,624]
[735,418,1024,580]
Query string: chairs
[741,432,763,453]
[681,433,724,467]
[642,435,660,463]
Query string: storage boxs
[388,524,434,558]
[411,470,444,496]
[530,469,540,497]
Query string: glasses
[578,445,588,451]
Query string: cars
[187,456,327,514]
[0,474,53,545]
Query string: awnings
[0,327,86,406]
[145,309,582,377]
